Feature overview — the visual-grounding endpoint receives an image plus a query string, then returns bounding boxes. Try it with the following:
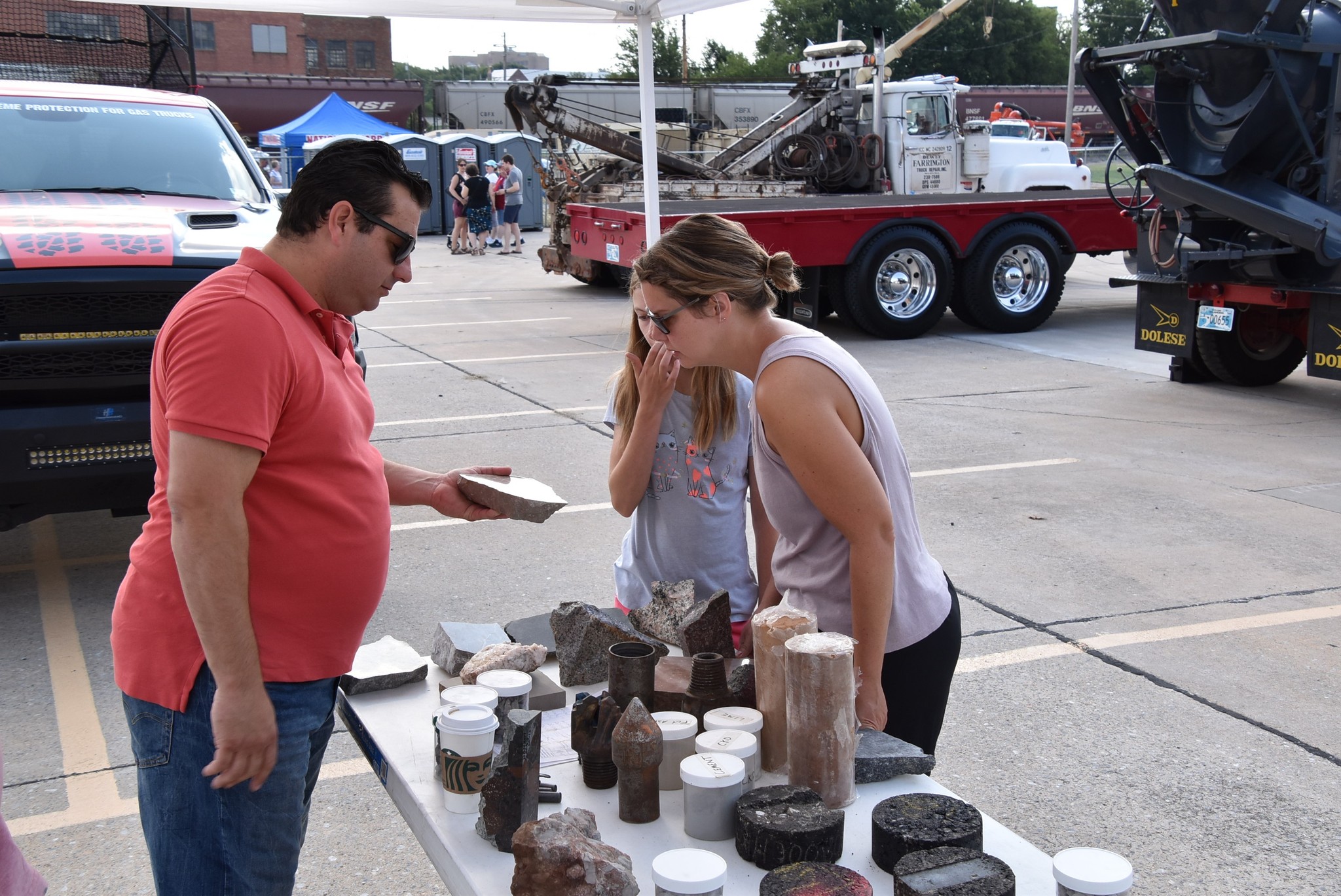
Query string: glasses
[458,164,466,167]
[348,204,417,265]
[485,164,492,167]
[645,294,710,335]
[498,166,502,169]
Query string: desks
[334,655,1057,896]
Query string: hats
[495,160,503,166]
[483,160,498,168]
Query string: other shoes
[489,239,503,247]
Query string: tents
[258,92,418,189]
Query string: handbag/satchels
[461,205,467,216]
[445,173,465,199]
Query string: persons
[603,269,781,656]
[109,140,513,896]
[449,153,525,255]
[256,155,284,189]
[636,213,962,777]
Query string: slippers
[510,250,522,254]
[496,249,510,255]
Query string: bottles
[431,703,461,784]
[695,729,757,794]
[651,847,727,896]
[680,752,745,842]
[650,711,698,790]
[440,685,498,715]
[703,706,764,782]
[476,669,532,745]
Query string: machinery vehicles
[1077,0,1341,384]
[507,39,1175,336]
[552,118,755,237]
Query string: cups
[435,703,500,814]
[609,642,655,712]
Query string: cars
[2,76,367,533]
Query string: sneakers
[486,237,495,244]
[511,238,525,246]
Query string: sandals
[479,249,485,255]
[471,247,478,255]
[450,248,466,255]
[461,248,472,254]
